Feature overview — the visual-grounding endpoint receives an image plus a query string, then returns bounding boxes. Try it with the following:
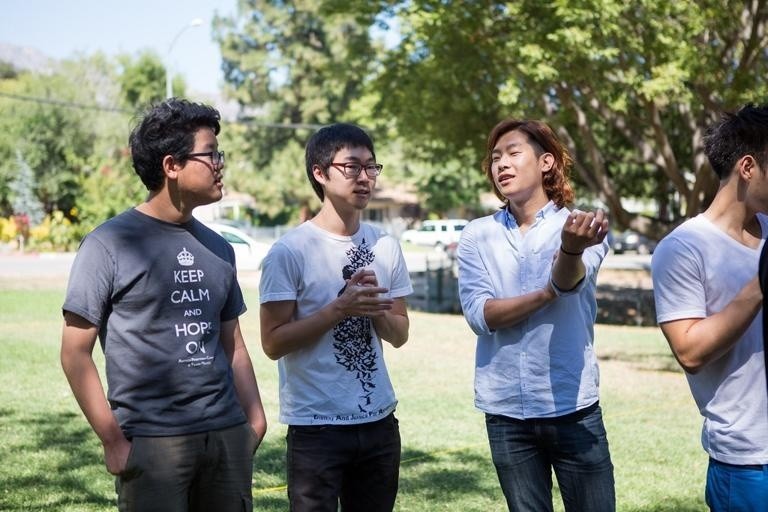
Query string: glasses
[328,162,383,178]
[180,150,225,165]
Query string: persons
[450,119,616,512]
[257,123,413,511]
[651,104,767,511]
[60,99,267,512]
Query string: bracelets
[559,245,585,255]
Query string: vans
[402,219,469,253]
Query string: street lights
[166,18,204,99]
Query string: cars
[613,229,657,254]
[205,223,271,270]
